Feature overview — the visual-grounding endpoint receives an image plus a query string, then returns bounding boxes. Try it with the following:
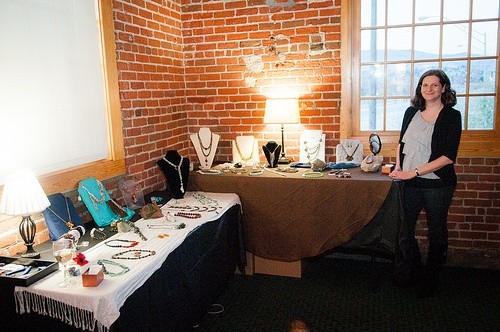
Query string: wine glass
[52,239,76,287]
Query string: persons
[388,69,462,297]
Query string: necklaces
[120,179,139,204]
[341,143,359,161]
[303,138,322,161]
[235,137,256,161]
[365,154,375,163]
[370,139,383,146]
[47,197,74,230]
[78,179,107,208]
[198,130,213,166]
[264,144,280,167]
[53,192,223,276]
[163,153,185,193]
[194,163,351,179]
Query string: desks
[186,160,404,277]
[0,190,244,332]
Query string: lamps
[265,98,300,163]
[0,166,52,259]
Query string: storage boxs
[82,265,105,287]
[382,162,395,174]
[255,255,307,278]
[236,251,255,276]
[0,256,59,288]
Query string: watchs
[415,168,420,177]
